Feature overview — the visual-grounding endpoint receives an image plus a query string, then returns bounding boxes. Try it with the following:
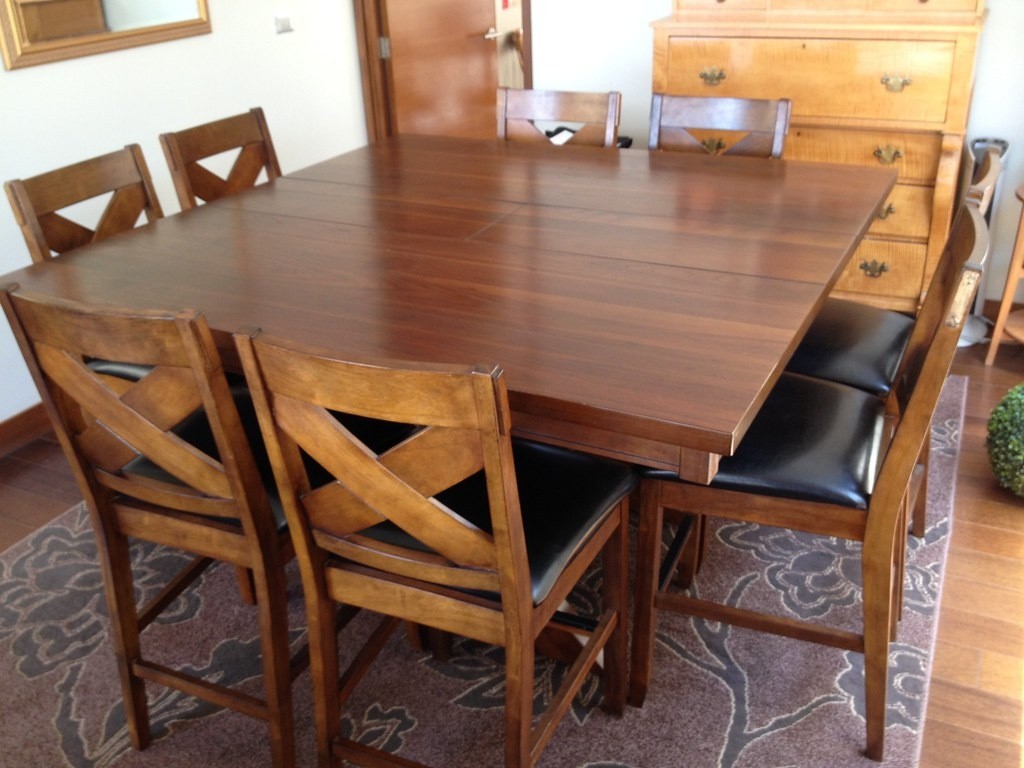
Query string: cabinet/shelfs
[983,180,1023,366]
[649,0,986,319]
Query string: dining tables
[0,132,897,645]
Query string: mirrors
[0,1,212,70]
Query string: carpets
[0,375,968,768]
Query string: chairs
[158,107,284,213]
[495,88,621,147]
[649,93,792,159]
[0,280,428,768]
[231,322,638,768]
[4,142,166,263]
[628,204,991,763]
[697,145,1000,622]
